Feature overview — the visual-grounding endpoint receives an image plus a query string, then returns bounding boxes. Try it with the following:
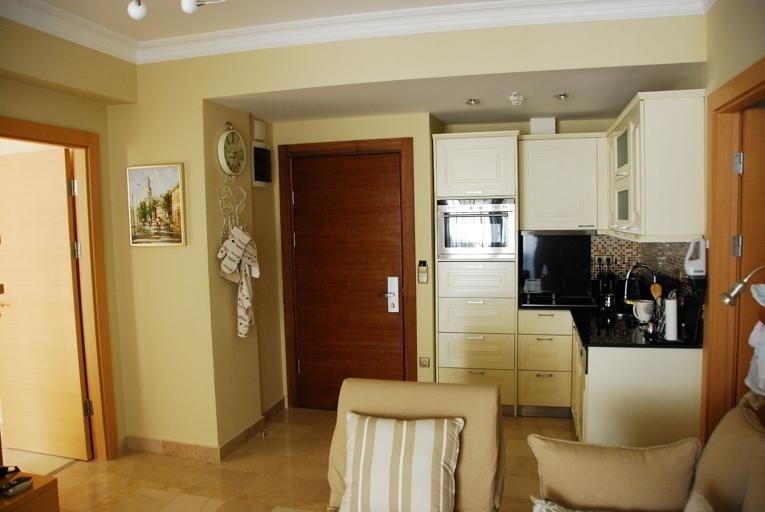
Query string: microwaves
[438,200,514,257]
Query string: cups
[632,325,657,345]
[632,299,655,324]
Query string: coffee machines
[601,293,616,316]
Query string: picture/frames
[125,161,187,248]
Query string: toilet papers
[662,298,679,342]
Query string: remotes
[2,476,33,497]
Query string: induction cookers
[521,293,597,308]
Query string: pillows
[527,491,585,511]
[683,489,713,511]
[524,429,704,512]
[334,406,467,512]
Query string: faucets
[623,262,659,301]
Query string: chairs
[325,374,510,512]
[518,381,764,512]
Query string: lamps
[126,0,229,23]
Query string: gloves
[216,227,251,275]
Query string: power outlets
[593,255,604,266]
[602,254,614,265]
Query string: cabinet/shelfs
[435,259,519,416]
[431,129,522,200]
[516,129,611,236]
[600,86,708,246]
[566,305,707,451]
[0,465,59,511]
[515,302,574,422]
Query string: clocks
[215,119,248,178]
